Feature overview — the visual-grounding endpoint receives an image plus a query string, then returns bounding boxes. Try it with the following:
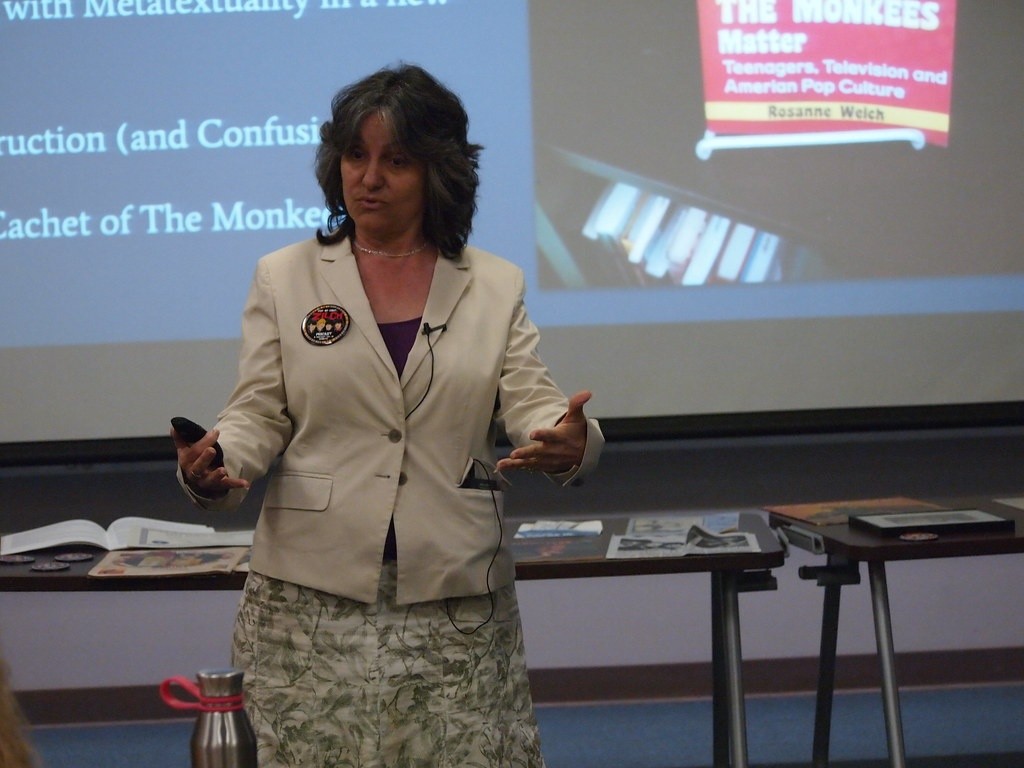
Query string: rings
[190,469,203,479]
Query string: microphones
[423,323,429,336]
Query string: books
[760,495,951,527]
[1,516,214,558]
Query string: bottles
[160,667,257,768]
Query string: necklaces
[353,241,430,257]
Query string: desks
[0,512,790,768]
[762,494,1024,768]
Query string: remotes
[171,417,224,459]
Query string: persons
[167,64,606,767]
